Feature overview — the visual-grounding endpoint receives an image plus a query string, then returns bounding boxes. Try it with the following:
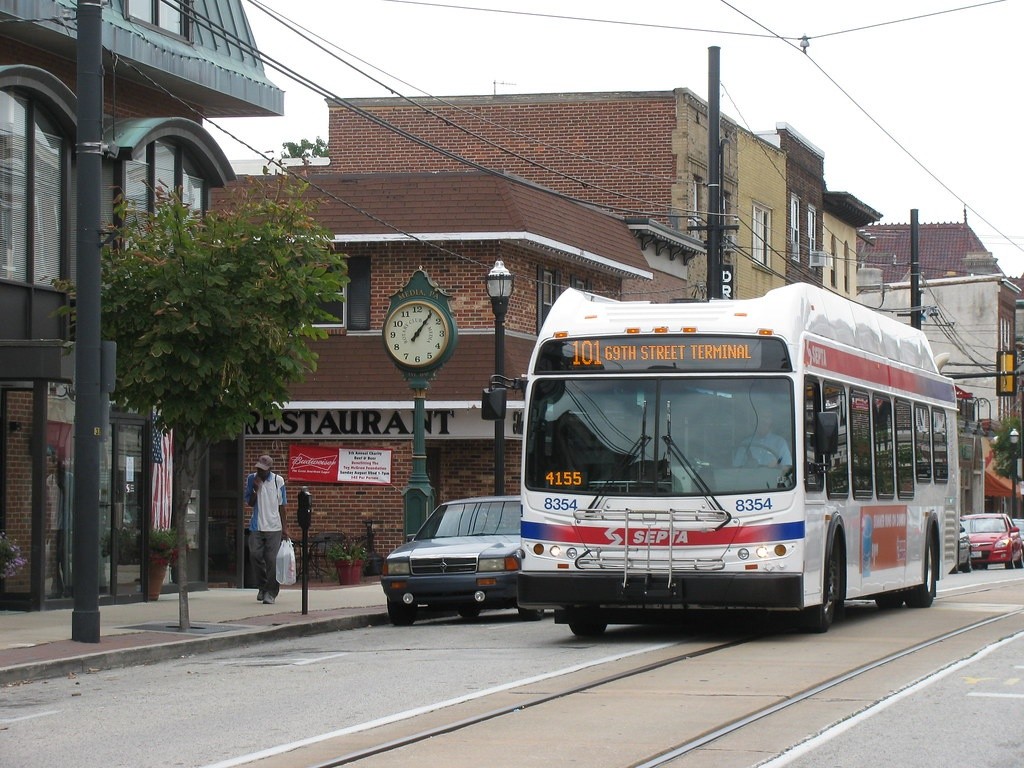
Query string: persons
[733,407,792,468]
[46,460,74,599]
[245,455,290,604]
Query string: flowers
[330,544,374,564]
[139,528,178,565]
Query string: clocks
[383,300,449,369]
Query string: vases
[147,564,170,600]
[330,560,370,585]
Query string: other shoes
[257,589,266,600]
[263,592,275,603]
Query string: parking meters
[294,488,313,615]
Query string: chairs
[288,537,337,583]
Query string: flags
[152,412,173,533]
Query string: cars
[950,519,972,573]
[381,491,553,626]
[1010,519,1024,544]
[961,513,1024,570]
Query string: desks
[298,538,333,581]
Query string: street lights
[1010,430,1020,518]
[487,260,514,495]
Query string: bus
[482,280,961,636]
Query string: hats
[254,454,273,470]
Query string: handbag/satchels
[275,538,296,585]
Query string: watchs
[253,488,256,494]
